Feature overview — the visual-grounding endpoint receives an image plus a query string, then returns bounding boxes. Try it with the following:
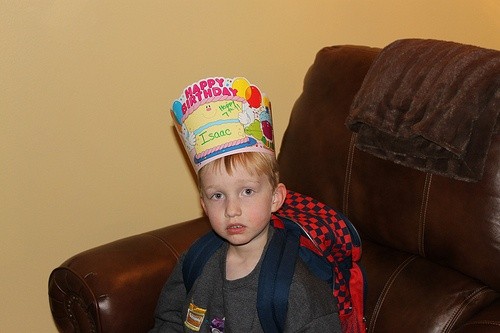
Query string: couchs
[48,38,500,333]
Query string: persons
[149,141,342,333]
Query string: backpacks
[183,189,367,333]
